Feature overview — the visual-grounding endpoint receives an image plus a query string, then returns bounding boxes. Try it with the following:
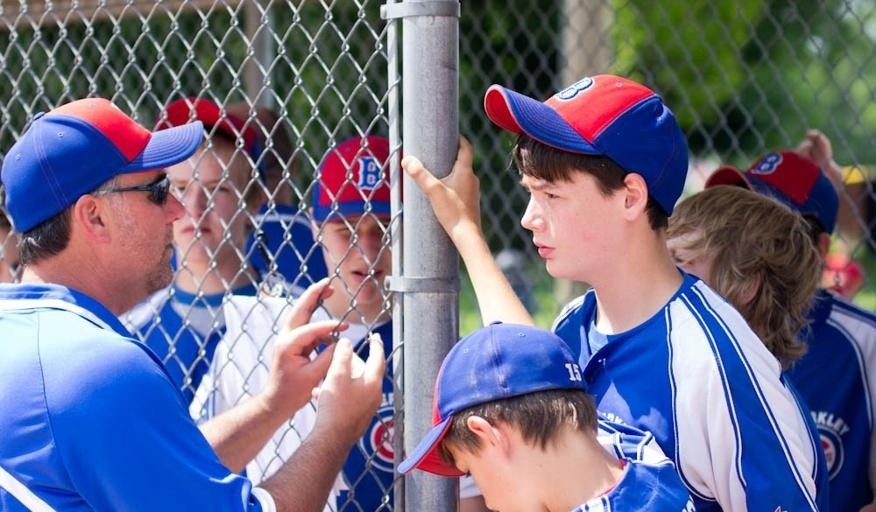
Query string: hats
[309,134,419,226]
[148,80,266,181]
[1,96,205,236]
[482,74,691,220]
[704,149,842,243]
[396,319,592,479]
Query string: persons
[399,74,876,512]
[0,72,395,511]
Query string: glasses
[80,177,175,209]
